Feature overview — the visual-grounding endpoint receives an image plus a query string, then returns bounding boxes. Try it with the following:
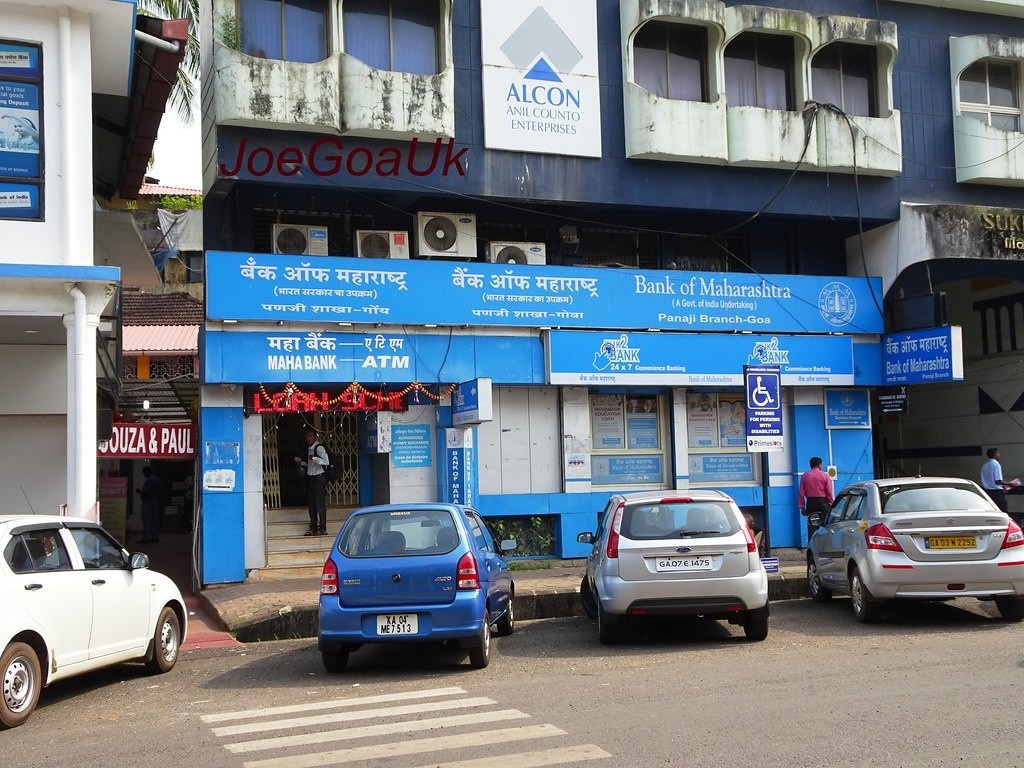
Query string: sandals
[310,529,326,536]
[304,530,319,536]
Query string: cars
[318,502,517,673]
[577,489,770,644]
[806,477,1024,624]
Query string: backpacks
[314,444,343,480]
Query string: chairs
[631,511,647,533]
[11,540,48,570]
[680,508,710,530]
[375,531,406,554]
[437,526,459,548]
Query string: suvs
[0,514,188,730]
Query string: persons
[134,467,159,542]
[293,429,330,535]
[798,457,835,541]
[980,447,1018,513]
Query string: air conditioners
[270,223,329,256]
[485,241,546,265]
[354,230,409,259]
[413,211,478,262]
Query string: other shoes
[136,540,147,543]
[149,539,158,542]
